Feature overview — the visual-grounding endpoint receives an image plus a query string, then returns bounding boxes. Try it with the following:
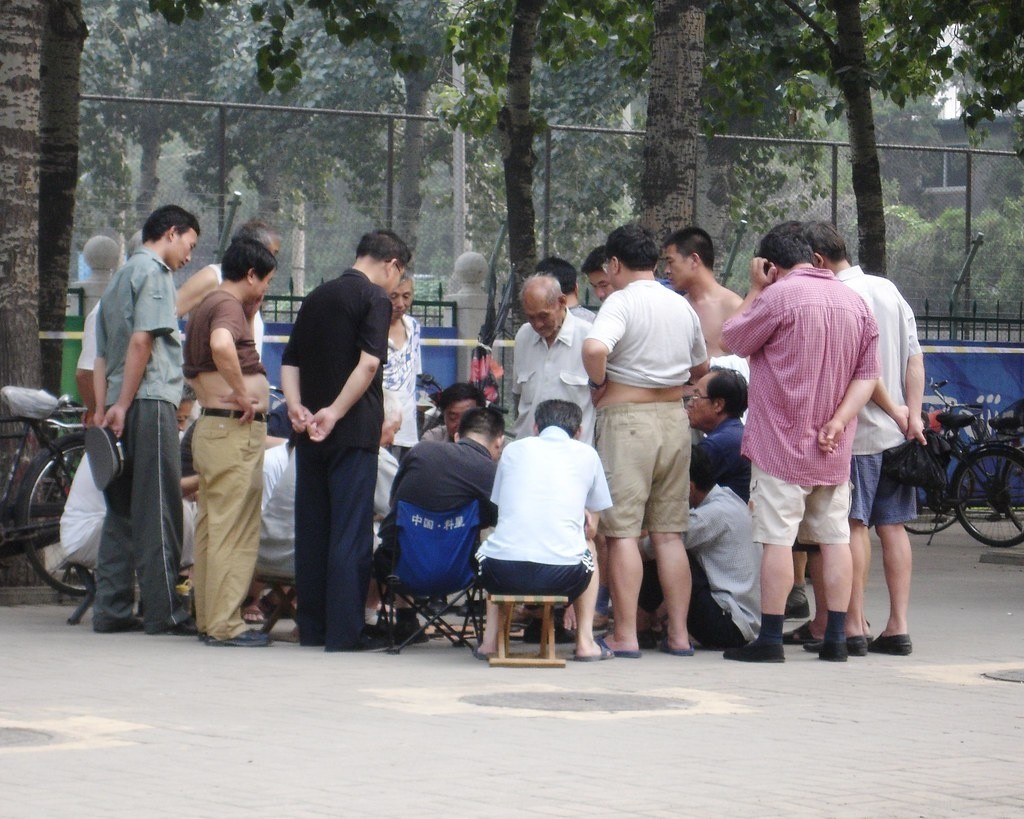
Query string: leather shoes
[198,628,270,646]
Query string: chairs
[377,498,483,654]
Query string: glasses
[689,392,710,406]
[601,262,612,274]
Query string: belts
[201,407,269,423]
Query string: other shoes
[524,623,577,644]
[152,618,199,635]
[868,631,912,655]
[819,640,847,662]
[637,628,655,650]
[864,634,876,652]
[722,640,785,663]
[783,600,811,618]
[802,640,823,652]
[344,629,391,651]
[593,613,610,631]
[95,618,146,633]
[392,626,429,645]
[846,636,867,656]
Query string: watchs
[589,372,608,390]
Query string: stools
[488,595,570,667]
[65,563,97,626]
[255,575,298,634]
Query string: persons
[473,399,616,662]
[581,224,709,659]
[720,223,880,662]
[181,237,271,647]
[92,203,199,636]
[58,222,828,645]
[804,221,930,656]
[280,228,413,651]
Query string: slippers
[241,605,266,624]
[659,634,696,656]
[574,641,615,662]
[782,620,824,644]
[262,594,297,618]
[595,638,641,659]
[472,642,500,660]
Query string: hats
[85,426,125,491]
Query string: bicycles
[0,384,110,627]
[900,379,1024,548]
